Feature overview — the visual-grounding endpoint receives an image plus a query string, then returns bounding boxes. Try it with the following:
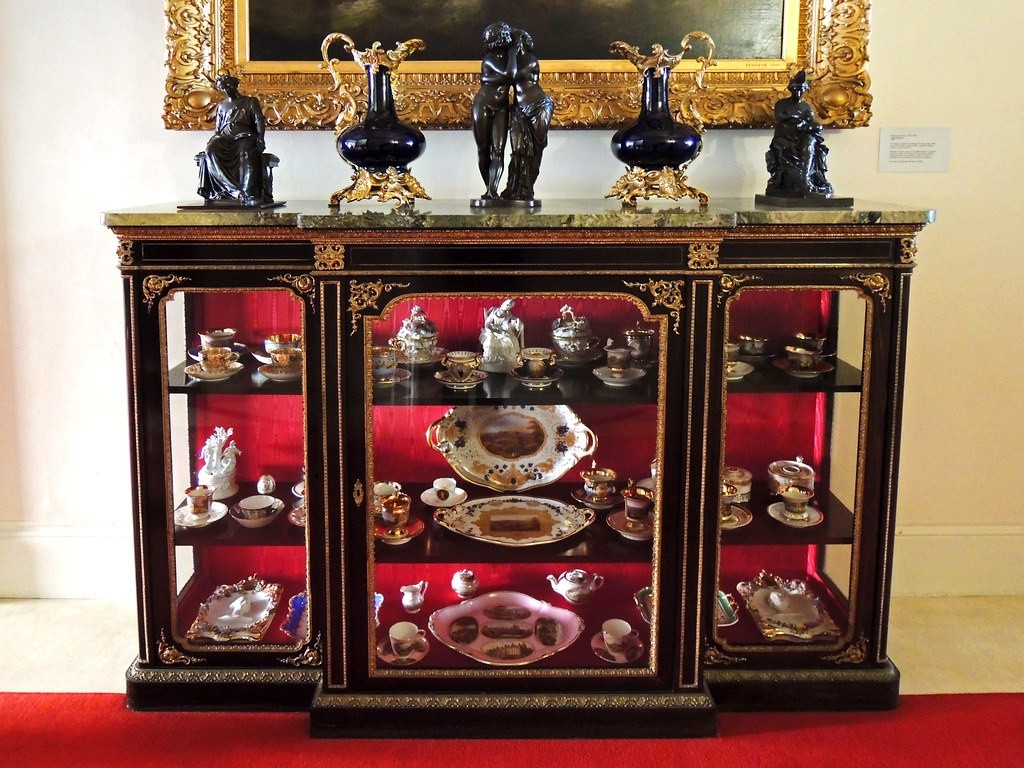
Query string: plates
[174,322,840,663]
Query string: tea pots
[452,567,477,600]
[547,568,604,606]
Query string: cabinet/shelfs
[100,209,936,739]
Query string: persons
[471,22,555,200]
[196,75,266,206]
[478,299,522,361]
[765,68,834,199]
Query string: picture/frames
[161,0,873,130]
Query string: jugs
[400,579,429,614]
